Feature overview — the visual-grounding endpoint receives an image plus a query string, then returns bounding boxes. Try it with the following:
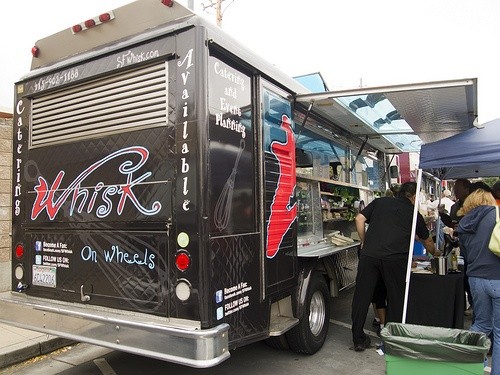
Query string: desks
[405,257,465,329]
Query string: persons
[458,188,500,375]
[438,177,500,316]
[351,181,442,352]
[357,183,401,339]
[425,189,456,256]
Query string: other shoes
[373,317,380,327]
[355,336,371,351]
[377,324,384,337]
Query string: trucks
[0,0,402,372]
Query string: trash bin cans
[379,321,492,375]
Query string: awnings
[294,78,478,182]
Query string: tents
[401,117,500,324]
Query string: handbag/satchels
[489,205,500,256]
